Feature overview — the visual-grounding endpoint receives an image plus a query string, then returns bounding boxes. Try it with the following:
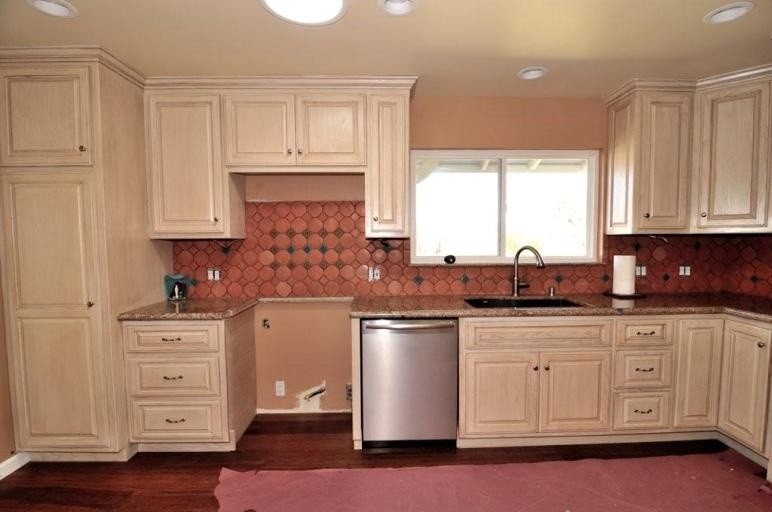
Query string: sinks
[465,294,586,309]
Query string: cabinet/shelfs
[220,76,365,173]
[606,63,771,234]
[457,316,607,448]
[0,47,174,462]
[144,77,246,240]
[121,307,254,451]
[608,313,771,484]
[365,76,417,238]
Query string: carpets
[215,448,772,512]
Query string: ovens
[360,319,459,456]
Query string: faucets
[512,245,545,297]
